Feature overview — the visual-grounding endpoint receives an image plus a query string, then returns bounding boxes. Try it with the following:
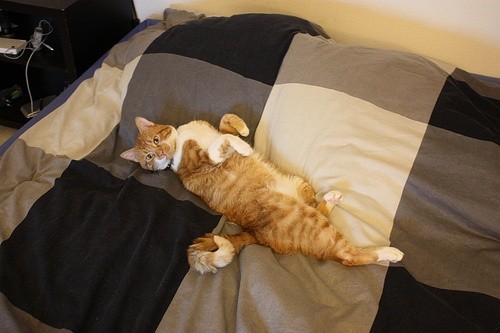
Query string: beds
[0,6,500,333]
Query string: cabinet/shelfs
[1,0,138,131]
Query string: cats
[120,113,410,275]
